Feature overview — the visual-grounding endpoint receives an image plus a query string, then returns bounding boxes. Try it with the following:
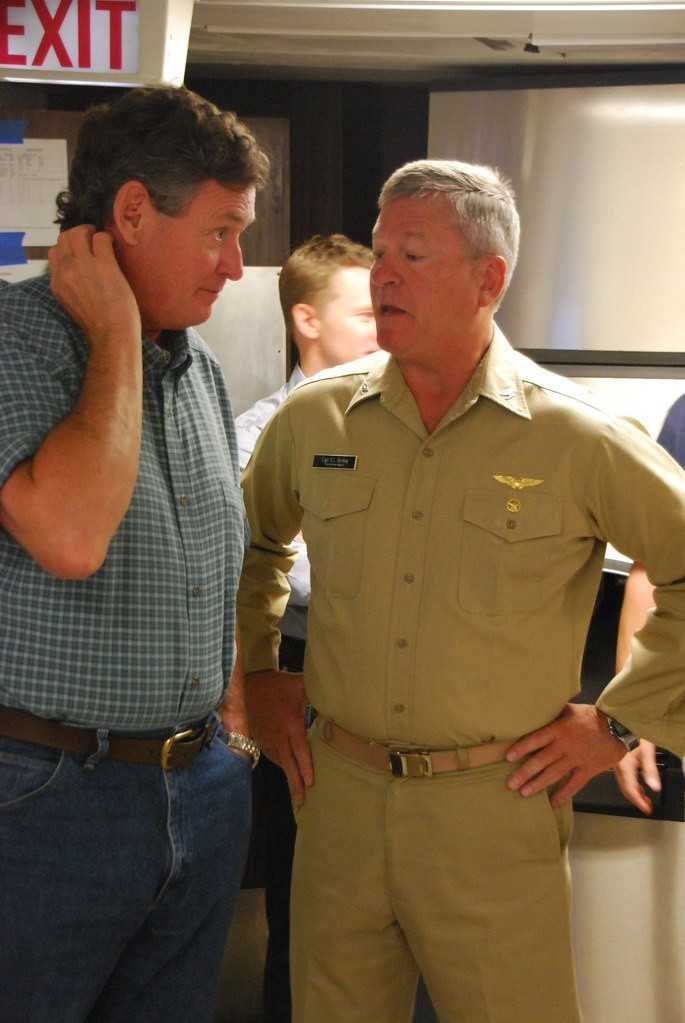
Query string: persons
[614,395,685,810]
[233,160,685,1023]
[0,83,258,1023]
[234,234,383,1020]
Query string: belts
[0,705,224,770]
[315,713,522,778]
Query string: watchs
[226,731,260,770]
[606,716,640,752]
